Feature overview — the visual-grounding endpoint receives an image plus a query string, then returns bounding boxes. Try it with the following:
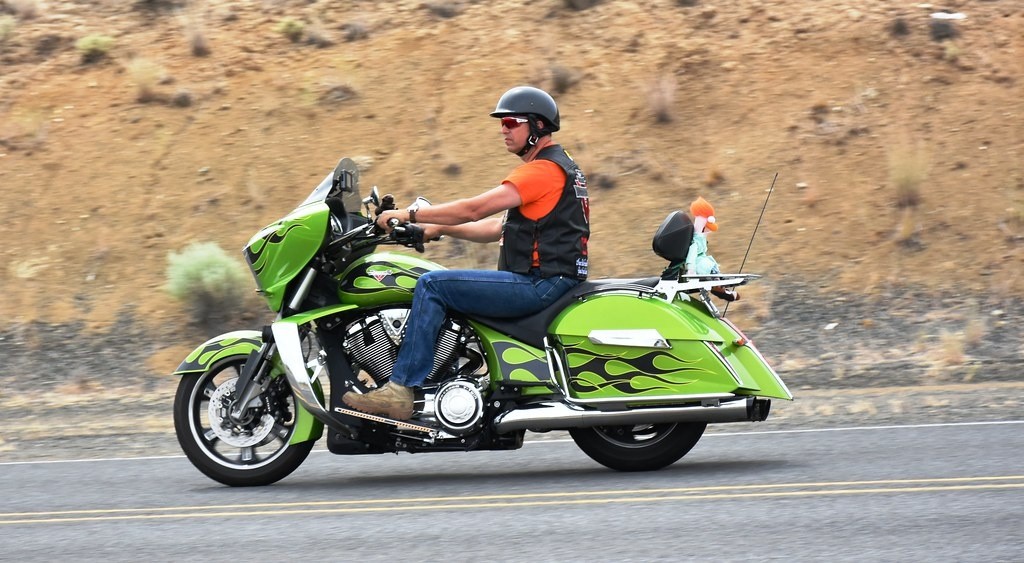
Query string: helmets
[489,86,560,132]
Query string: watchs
[407,203,419,222]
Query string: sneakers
[342,381,414,420]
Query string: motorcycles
[171,157,793,489]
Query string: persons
[342,87,589,419]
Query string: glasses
[500,117,528,129]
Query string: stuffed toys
[686,196,740,301]
